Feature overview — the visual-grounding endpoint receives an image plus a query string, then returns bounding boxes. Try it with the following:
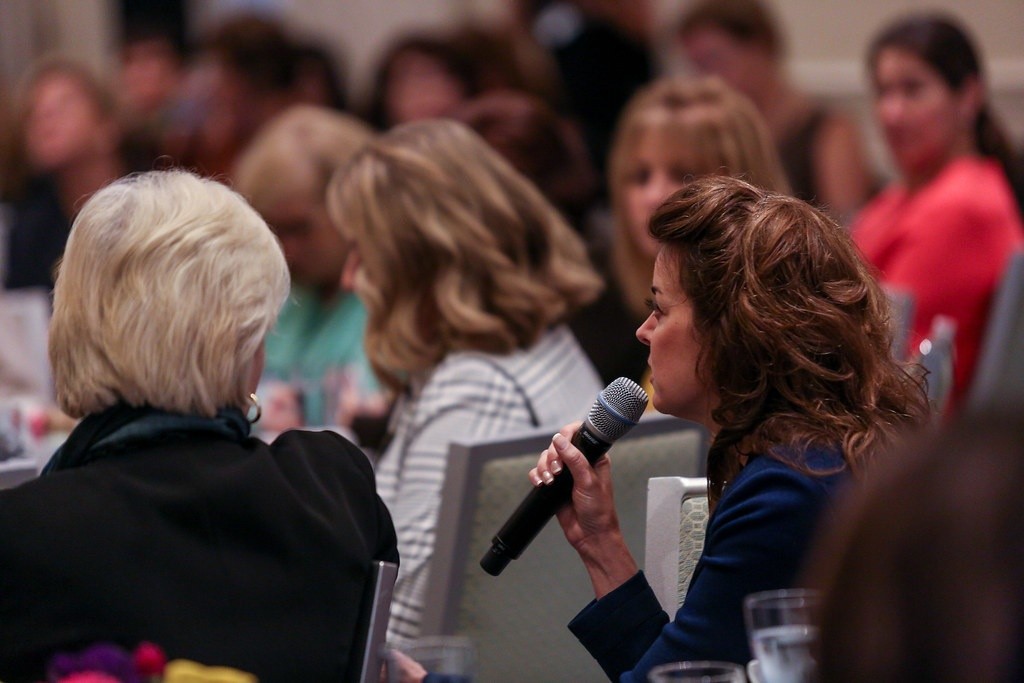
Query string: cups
[744,586,827,683]
[381,636,480,683]
[645,661,746,683]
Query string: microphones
[480,376,649,576]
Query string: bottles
[910,315,953,427]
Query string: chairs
[358,255,1023,682]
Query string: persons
[0,0,1024,683]
